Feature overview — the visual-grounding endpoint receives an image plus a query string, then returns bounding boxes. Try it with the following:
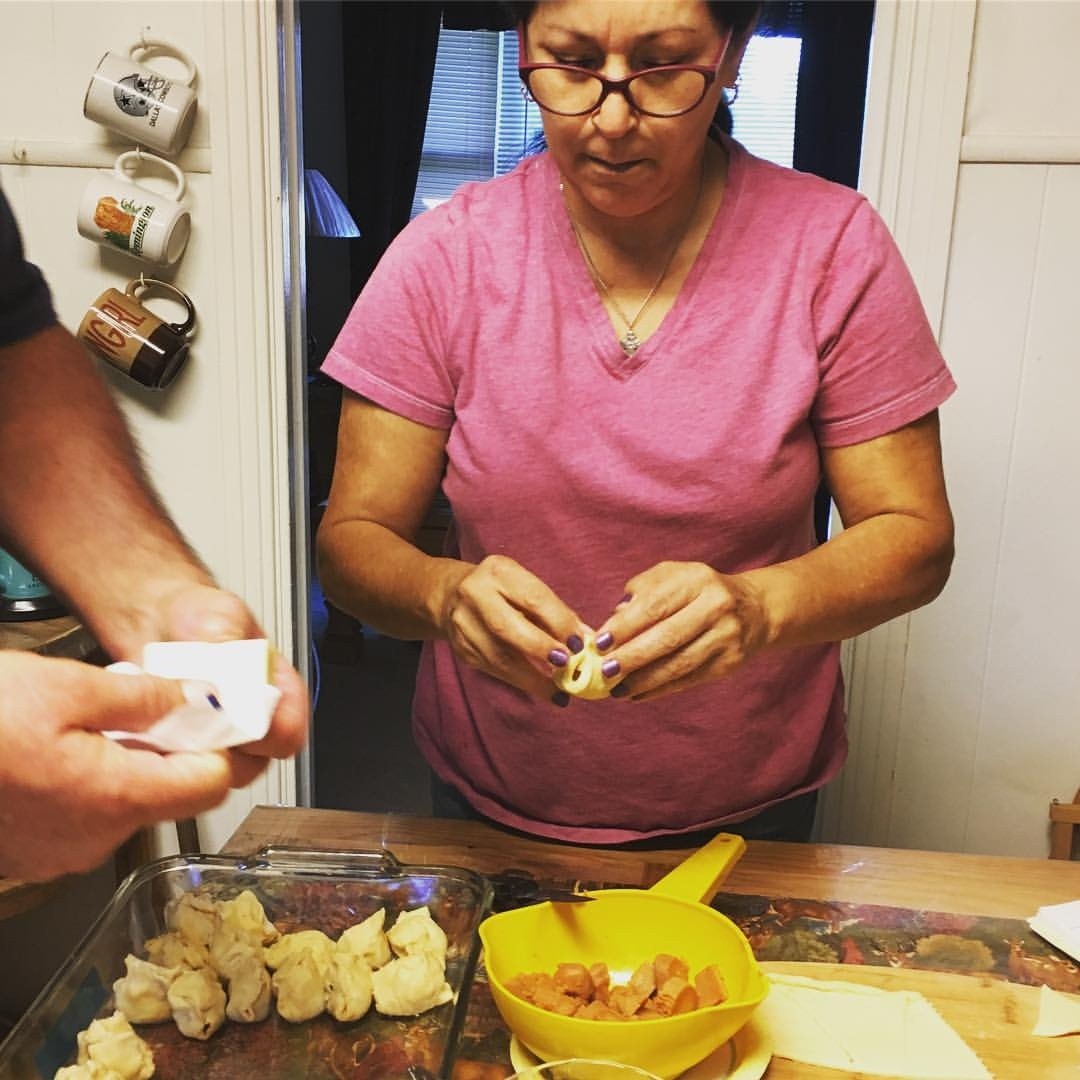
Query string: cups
[83,38,202,155]
[76,150,191,266]
[75,279,197,390]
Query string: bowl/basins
[1,846,496,1080]
[478,889,770,1078]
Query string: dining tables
[86,799,1080,1080]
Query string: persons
[1,185,310,890]
[320,0,958,848]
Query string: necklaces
[557,145,709,358]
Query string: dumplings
[60,881,457,1080]
[560,619,615,700]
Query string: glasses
[516,16,736,117]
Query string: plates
[510,1001,774,1079]
